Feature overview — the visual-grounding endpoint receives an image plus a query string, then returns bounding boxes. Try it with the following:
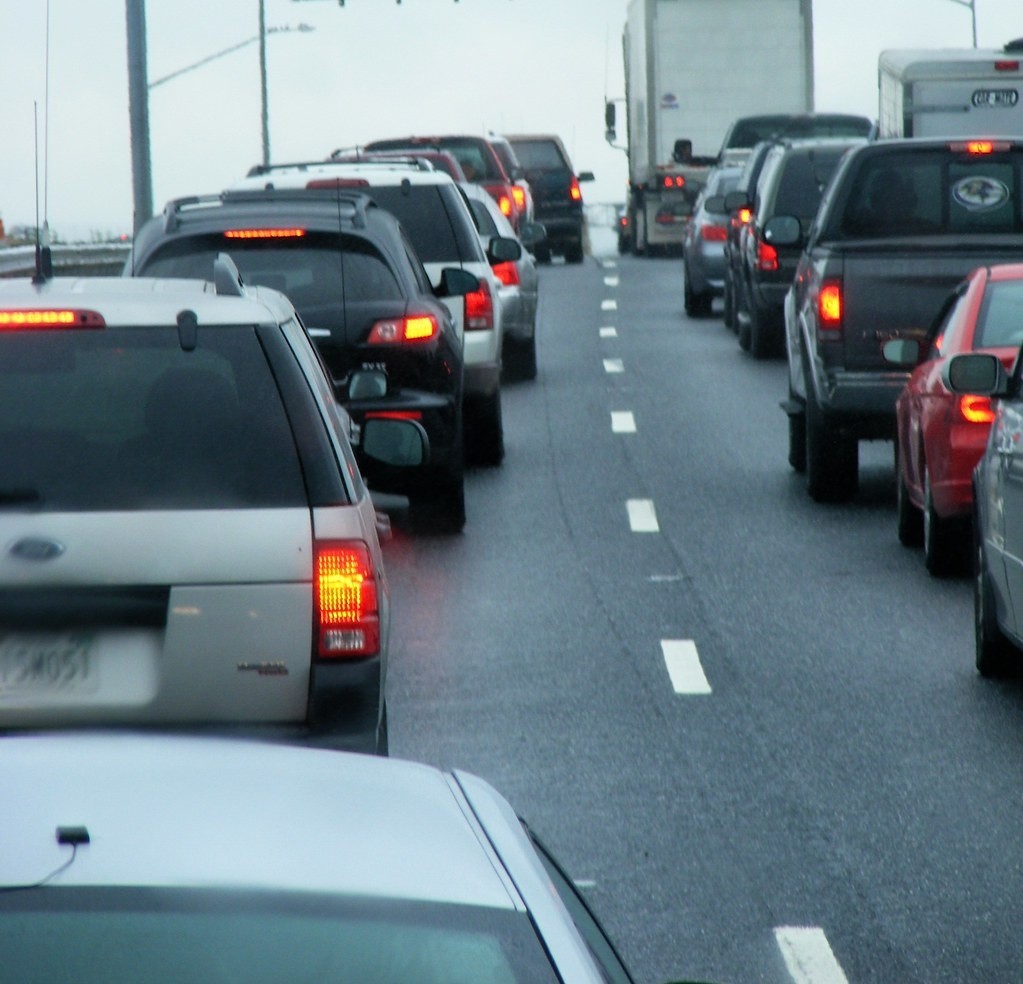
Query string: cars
[936,333,1023,681]
[873,257,1023,573]
[673,111,871,358]
[1,732,640,984]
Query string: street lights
[125,21,320,248]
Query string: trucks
[603,0,818,257]
[862,46,1023,146]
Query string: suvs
[206,129,596,464]
[121,188,481,537]
[761,135,1023,502]
[0,248,433,772]
